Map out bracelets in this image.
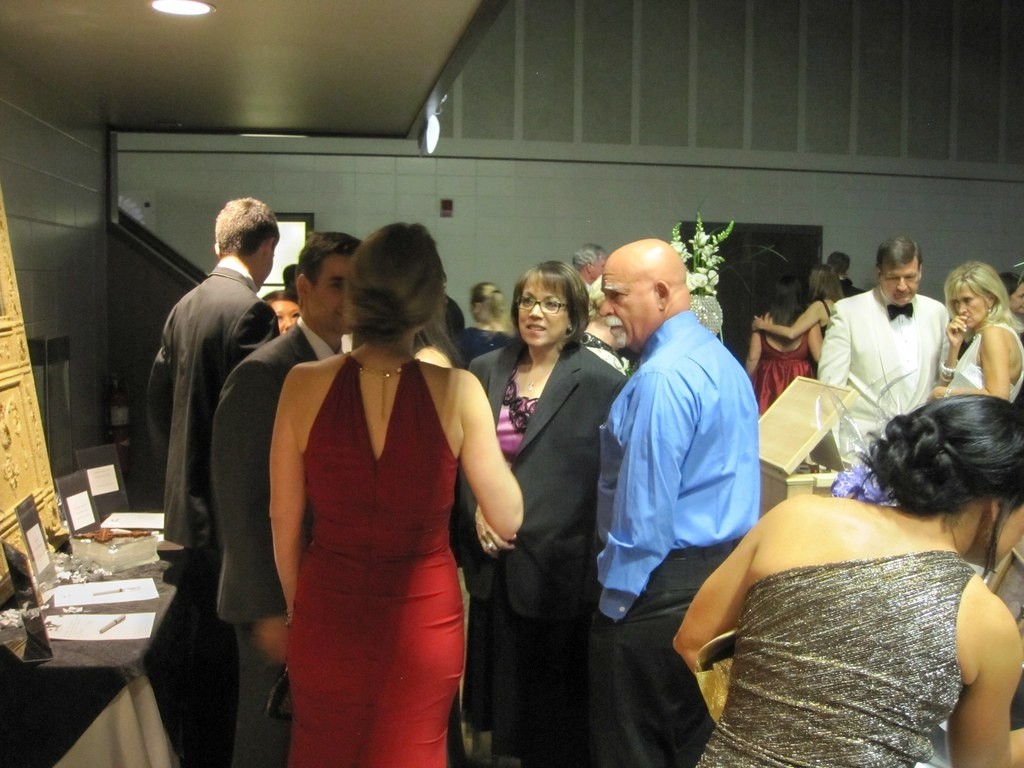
[941,360,958,382]
[944,389,951,397]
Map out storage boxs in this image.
[755,374,865,513]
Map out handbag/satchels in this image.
[693,628,739,725]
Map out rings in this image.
[486,542,494,550]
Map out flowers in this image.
[672,215,734,292]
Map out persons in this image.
[461,261,626,768]
[817,237,952,470]
[927,262,1024,403]
[148,199,279,767]
[415,270,513,768]
[262,265,301,336]
[673,394,1024,768]
[747,252,867,416]
[591,238,760,768]
[958,273,1024,360]
[573,246,627,378]
[214,231,361,768]
[270,223,523,768]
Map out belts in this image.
[667,539,740,559]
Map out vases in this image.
[687,289,726,336]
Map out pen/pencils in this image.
[99,614,126,634]
[93,587,144,597]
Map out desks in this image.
[0,504,184,768]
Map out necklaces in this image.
[526,364,550,391]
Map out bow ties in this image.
[887,302,914,320]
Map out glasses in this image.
[515,295,569,315]
